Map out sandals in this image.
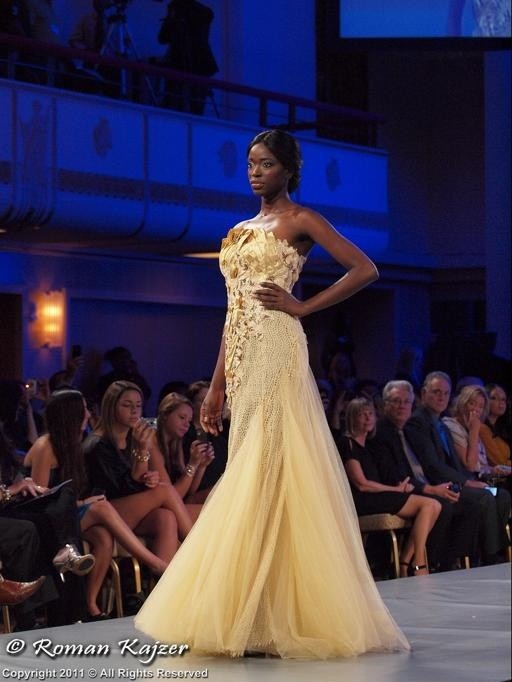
[390,560,427,578]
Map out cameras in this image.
[195,441,210,453]
[140,416,158,430]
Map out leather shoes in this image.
[1,571,48,608]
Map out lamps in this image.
[27,286,67,351]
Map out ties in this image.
[397,429,427,485]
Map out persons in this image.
[0,344,510,629]
[0,1,219,117]
[130,128,409,661]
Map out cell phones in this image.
[449,480,463,493]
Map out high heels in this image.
[53,543,99,584]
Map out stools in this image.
[0,508,512,637]
